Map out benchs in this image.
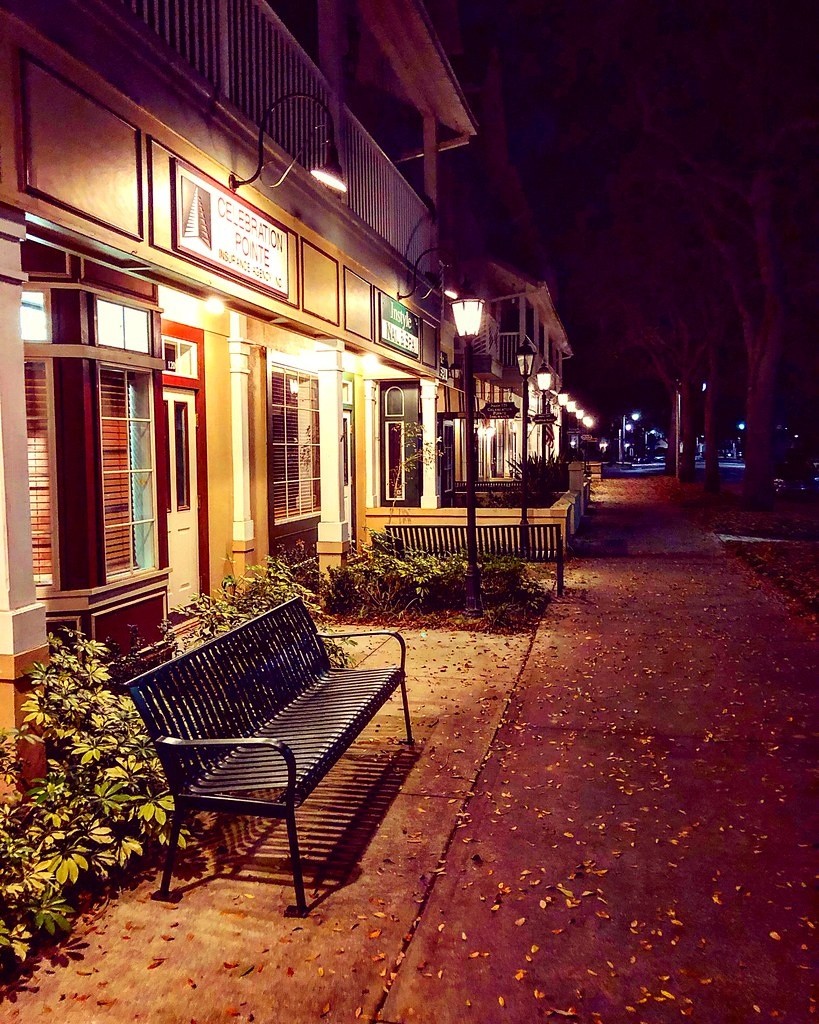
[385,523,568,595]
[123,595,416,917]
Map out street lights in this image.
[536,363,552,468]
[449,288,486,619]
[557,388,594,471]
[514,337,538,560]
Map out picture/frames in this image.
[145,133,301,311]
[421,318,438,370]
[17,46,145,243]
[343,265,374,342]
[373,285,422,363]
[300,236,340,328]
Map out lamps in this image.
[229,92,348,195]
[398,247,494,338]
[448,362,460,380]
[289,379,299,401]
